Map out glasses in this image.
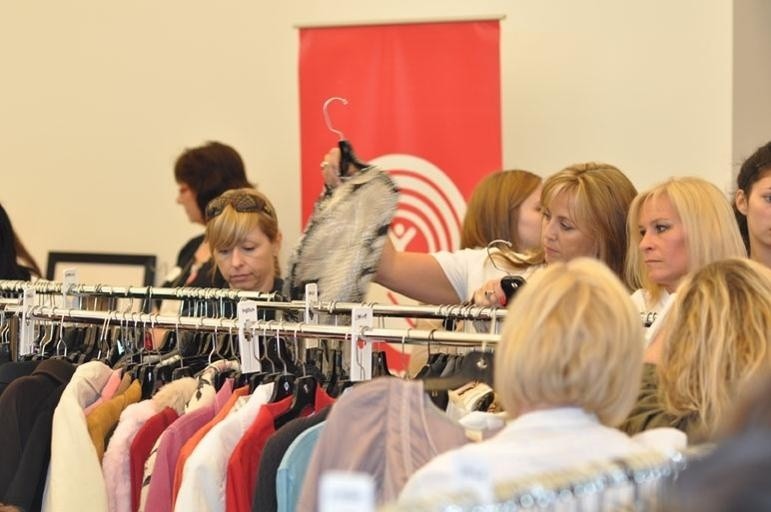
[204,192,275,222]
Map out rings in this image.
[321,162,330,169]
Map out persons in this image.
[1,203,42,300]
[394,261,693,509]
[458,169,545,356]
[321,145,638,311]
[621,176,747,333]
[616,262,771,427]
[151,139,255,305]
[180,188,289,354]
[733,140,771,267]
